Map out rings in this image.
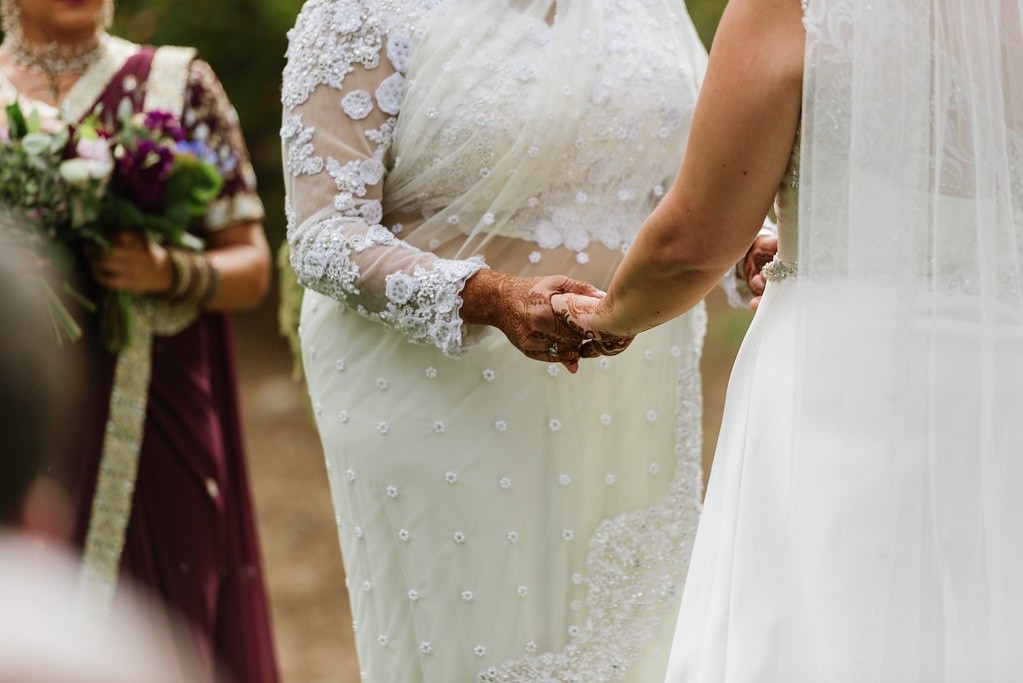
[544,342,560,357]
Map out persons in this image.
[281,0,783,683]
[0,0,281,683]
[0,214,226,682]
[548,0,1021,682]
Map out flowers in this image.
[2,91,240,247]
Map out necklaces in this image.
[1,31,105,73]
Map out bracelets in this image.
[149,248,217,313]
